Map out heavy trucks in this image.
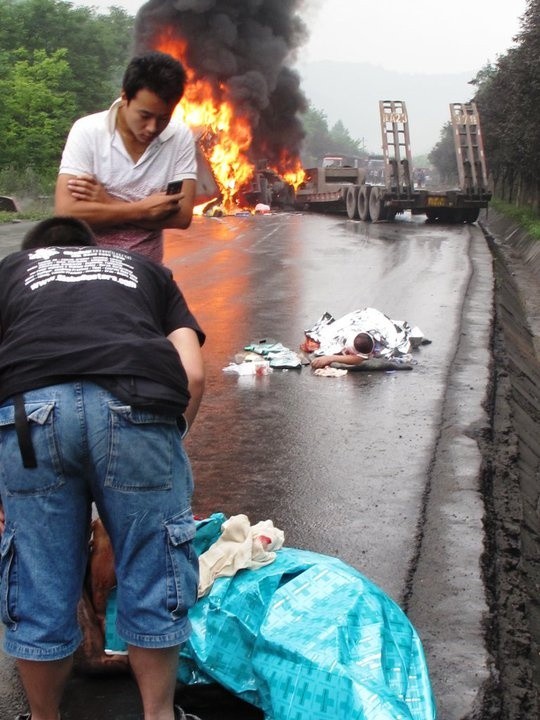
[294,99,492,223]
[365,157,395,185]
[318,152,365,169]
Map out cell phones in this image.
[166,180,183,195]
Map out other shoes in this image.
[15,710,32,720]
[173,703,202,720]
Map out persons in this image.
[52,52,197,267]
[312,307,397,369]
[0,486,435,720]
[0,216,206,720]
[366,165,429,188]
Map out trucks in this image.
[245,157,293,208]
